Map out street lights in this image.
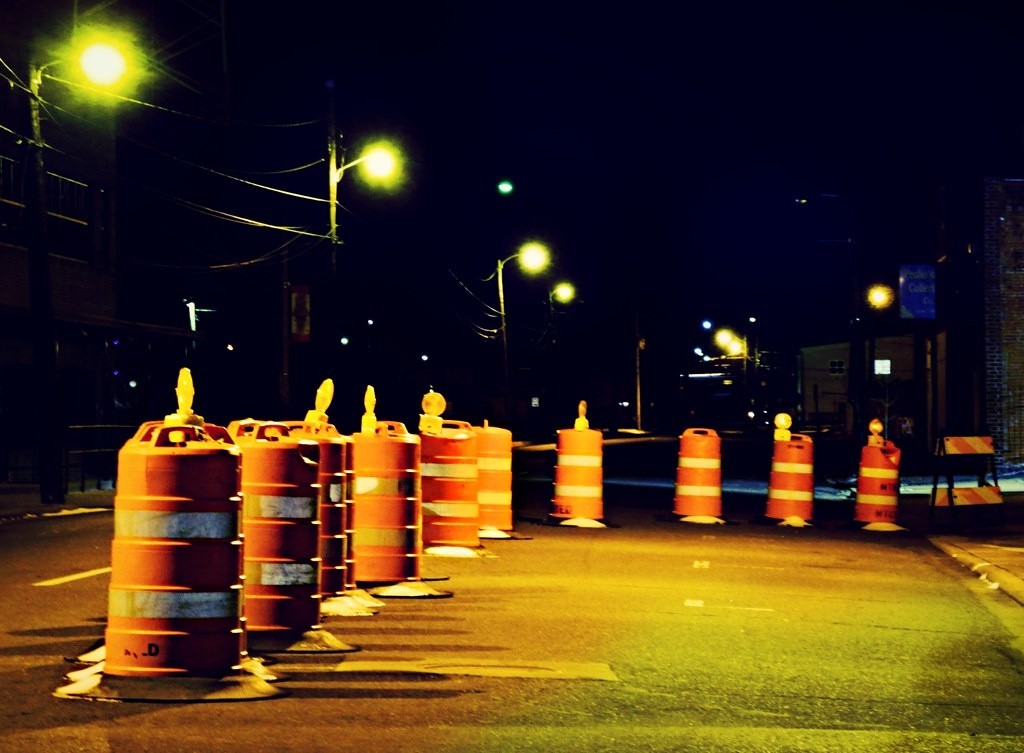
[494,241,553,350]
[326,107,409,248]
[19,21,146,152]
